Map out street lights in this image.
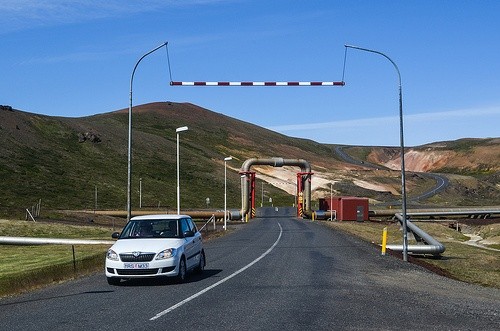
[224,157,233,230]
[176,126,188,215]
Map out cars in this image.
[105,215,207,284]
